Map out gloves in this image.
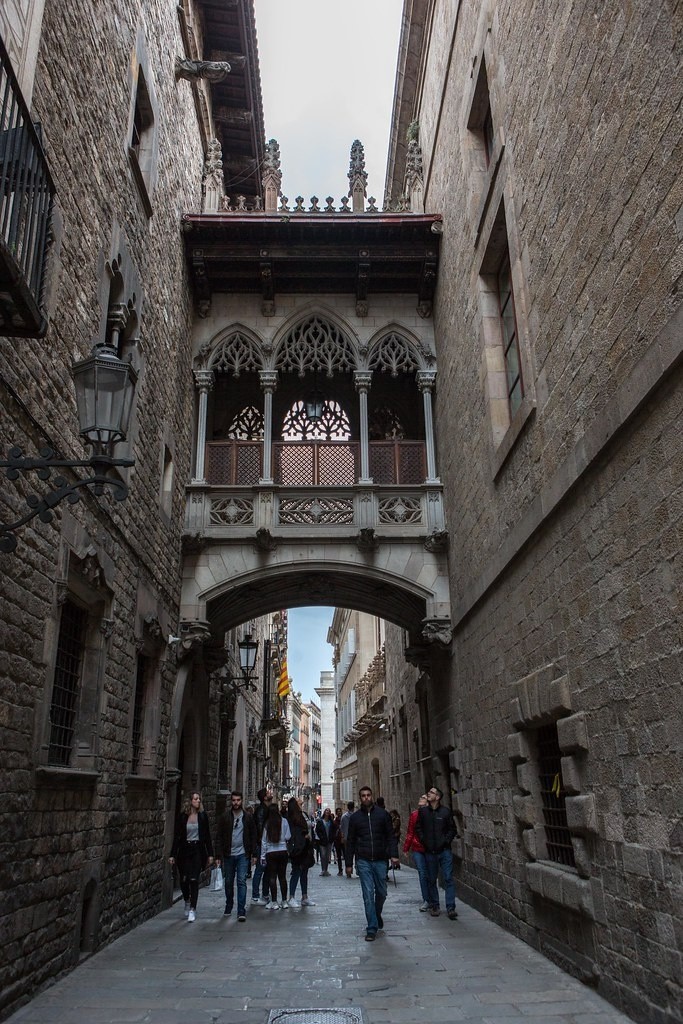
[260,859,267,867]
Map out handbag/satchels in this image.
[208,863,222,892]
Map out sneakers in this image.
[188,910,196,922]
[251,896,316,910]
[184,905,191,916]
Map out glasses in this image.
[235,818,239,827]
[429,791,437,795]
[421,796,428,800]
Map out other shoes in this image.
[376,913,383,929]
[447,906,458,917]
[430,903,440,917]
[365,932,376,941]
[246,873,251,878]
[319,871,331,876]
[419,900,433,912]
[224,905,232,915]
[239,915,247,921]
[347,873,351,878]
[337,871,343,876]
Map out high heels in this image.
[395,864,400,870]
[389,865,395,870]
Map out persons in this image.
[213,791,258,921]
[377,797,401,870]
[168,791,214,922]
[245,788,354,910]
[346,786,400,941]
[403,787,458,917]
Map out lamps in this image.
[0,344,134,557]
[210,636,262,705]
